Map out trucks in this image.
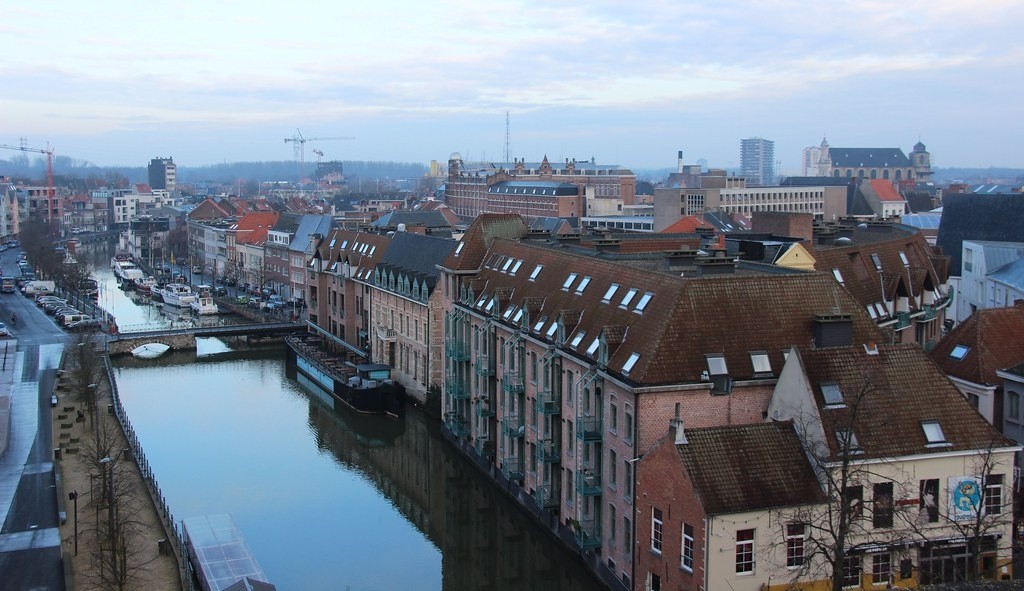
[0,276,15,293]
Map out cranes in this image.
[0,137,53,155]
[283,128,356,182]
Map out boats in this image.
[181,513,276,590]
[283,330,405,418]
[111,249,222,316]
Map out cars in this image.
[33,291,93,328]
[239,282,306,313]
[155,262,187,283]
[191,265,203,274]
[206,284,228,297]
[15,250,36,284]
[0,240,17,253]
[0,322,8,336]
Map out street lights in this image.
[99,455,118,583]
[87,383,99,454]
